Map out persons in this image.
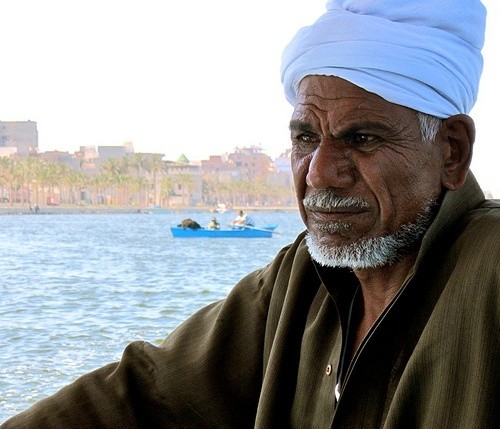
[0,0,499,429]
[208,217,220,229]
[230,209,255,230]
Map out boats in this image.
[137,206,173,215]
[210,204,234,214]
[171,224,277,238]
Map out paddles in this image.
[226,223,281,234]
